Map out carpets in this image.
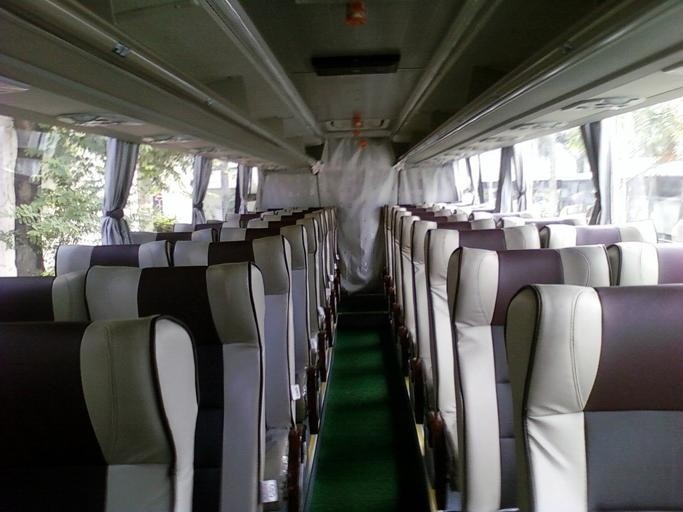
[301,280,437,512]
